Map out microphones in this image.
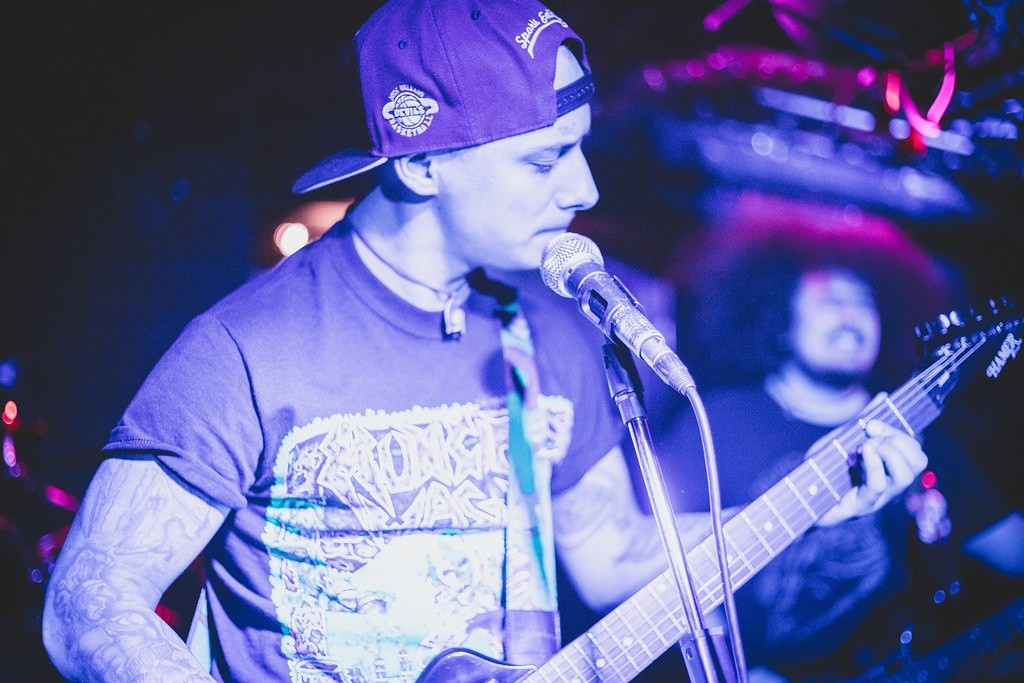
[539,232,696,396]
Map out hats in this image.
[291,0,595,196]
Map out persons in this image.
[36,0,931,682]
[652,267,955,683]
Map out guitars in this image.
[410,293,1023,683]
